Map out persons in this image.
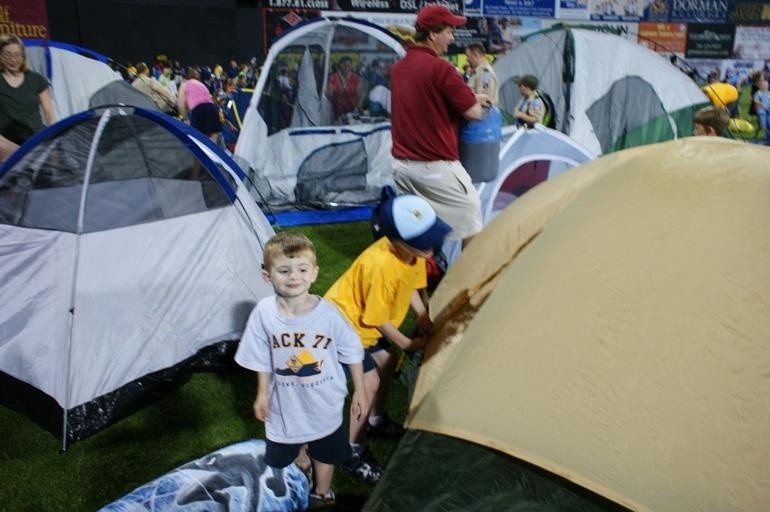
[387,5,493,255]
[111,53,470,153]
[319,191,454,484]
[461,63,473,84]
[0,31,57,164]
[511,74,557,131]
[462,41,500,109]
[669,53,770,143]
[234,226,371,511]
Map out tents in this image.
[491,21,745,158]
[356,136,770,510]
[21,37,188,138]
[432,118,598,275]
[230,14,415,236]
[2,100,277,456]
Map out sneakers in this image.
[343,460,383,483]
[310,493,336,510]
[370,411,405,435]
[294,463,314,490]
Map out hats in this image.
[513,73,538,89]
[414,6,467,30]
[378,195,449,250]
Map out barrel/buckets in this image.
[457,102,502,184]
